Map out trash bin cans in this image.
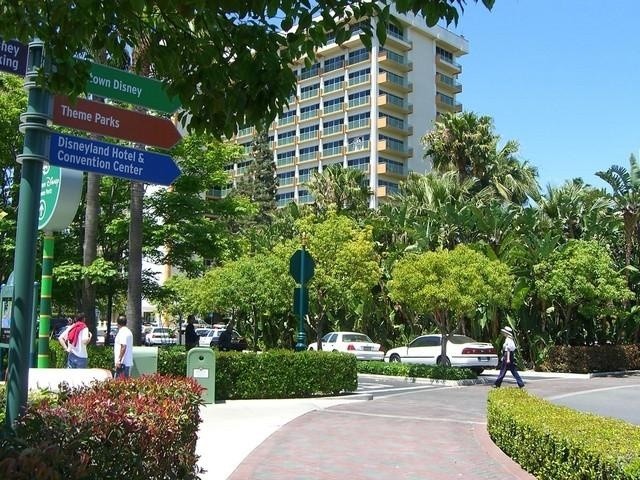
[186,348,215,404]
[130,347,158,377]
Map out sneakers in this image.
[493,385,500,389]
[520,385,526,389]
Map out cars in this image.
[380,332,500,378]
[34,311,250,355]
[305,330,385,361]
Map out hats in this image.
[501,325,513,336]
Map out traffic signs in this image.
[45,87,184,151]
[0,34,29,75]
[38,133,181,191]
[49,50,186,118]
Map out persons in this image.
[58,312,93,368]
[492,326,525,388]
[185,315,200,351]
[218,326,235,352]
[114,316,134,378]
